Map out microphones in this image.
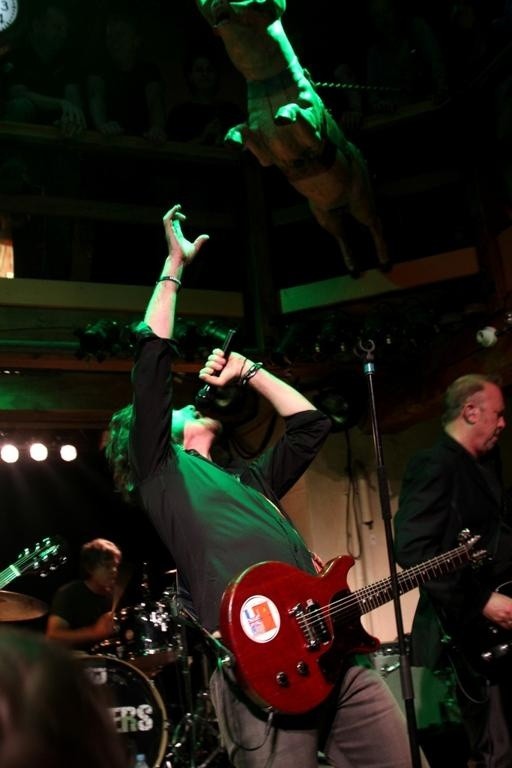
[194,327,241,409]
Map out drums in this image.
[92,602,186,674]
[165,694,234,768]
[69,652,168,768]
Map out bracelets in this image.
[239,357,248,378]
[237,361,264,386]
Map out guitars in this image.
[481,579,512,681]
[216,526,488,718]
[0,536,67,590]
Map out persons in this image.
[0,0,512,293]
[393,366,512,768]
[46,537,124,652]
[100,204,430,768]
